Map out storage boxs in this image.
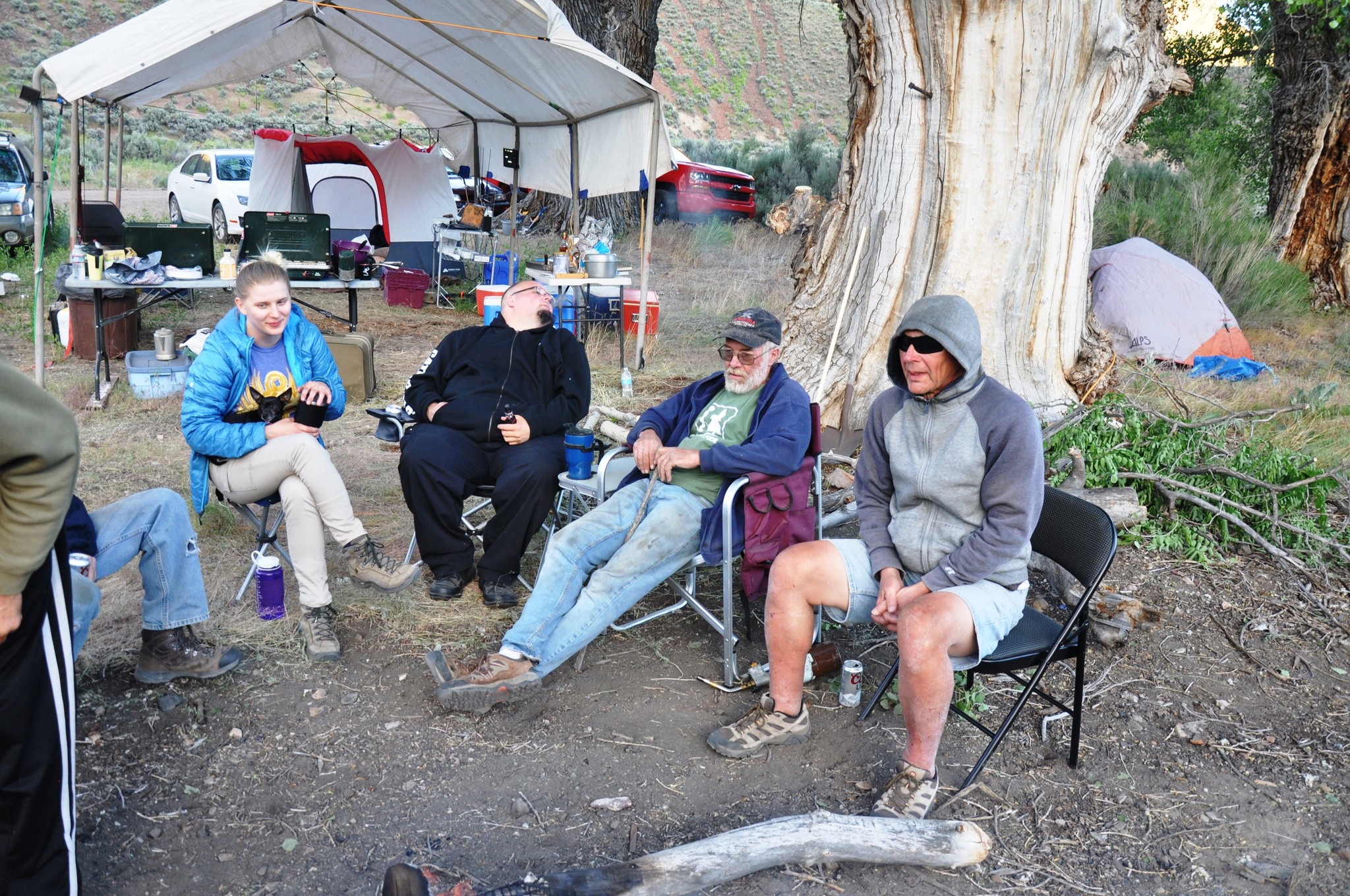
[476,284,659,337]
[236,211,331,279]
[125,351,192,400]
[124,221,216,273]
[423,292,436,303]
[381,266,429,308]
[69,297,139,360]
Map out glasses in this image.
[511,285,557,304]
[896,332,945,354]
[718,344,777,365]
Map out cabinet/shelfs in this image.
[432,224,499,311]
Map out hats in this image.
[712,307,783,346]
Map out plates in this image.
[169,277,202,280]
[1,275,20,281]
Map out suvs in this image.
[0,130,55,247]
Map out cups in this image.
[87,248,103,280]
[358,263,377,280]
[565,423,594,480]
[294,393,328,428]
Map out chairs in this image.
[363,406,559,597]
[858,485,1117,790]
[216,429,325,601]
[557,402,826,687]
[81,200,128,250]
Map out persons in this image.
[706,295,1045,819]
[424,308,814,712]
[397,283,590,608]
[180,261,421,661]
[64,488,243,685]
[1,358,82,896]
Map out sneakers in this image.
[869,758,940,819]
[341,534,421,593]
[134,626,243,683]
[705,692,811,760]
[423,649,490,716]
[435,651,542,713]
[296,603,343,661]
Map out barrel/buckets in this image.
[502,221,518,235]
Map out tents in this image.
[35,0,690,383]
[1087,237,1256,368]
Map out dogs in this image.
[200,385,293,467]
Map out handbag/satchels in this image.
[578,215,614,250]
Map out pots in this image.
[583,253,621,278]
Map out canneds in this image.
[839,659,863,706]
[69,552,90,580]
[384,404,402,419]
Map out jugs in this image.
[154,328,177,360]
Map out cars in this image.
[369,142,509,214]
[166,148,257,242]
[481,144,758,227]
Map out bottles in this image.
[568,235,580,273]
[504,404,516,424]
[251,550,285,621]
[72,245,85,281]
[219,249,237,280]
[621,368,633,398]
[93,239,102,249]
[553,252,569,278]
[559,232,568,252]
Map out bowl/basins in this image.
[331,240,371,263]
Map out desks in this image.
[64,272,381,413]
[525,268,632,371]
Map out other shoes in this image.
[478,576,517,608]
[382,864,430,896]
[428,564,476,601]
